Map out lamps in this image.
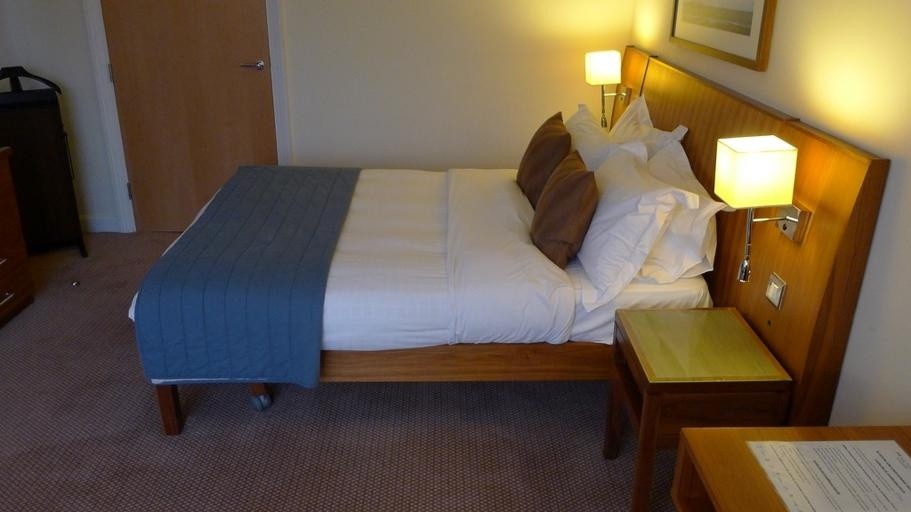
[584,50,633,127]
[713,135,811,284]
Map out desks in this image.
[670,424,909,512]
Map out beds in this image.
[128,45,890,434]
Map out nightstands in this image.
[603,306,796,511]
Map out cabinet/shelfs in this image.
[1,146,35,327]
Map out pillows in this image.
[529,149,597,269]
[608,92,689,162]
[577,146,699,313]
[516,111,571,212]
[633,143,727,284]
[563,104,620,172]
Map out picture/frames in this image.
[669,0,778,72]
